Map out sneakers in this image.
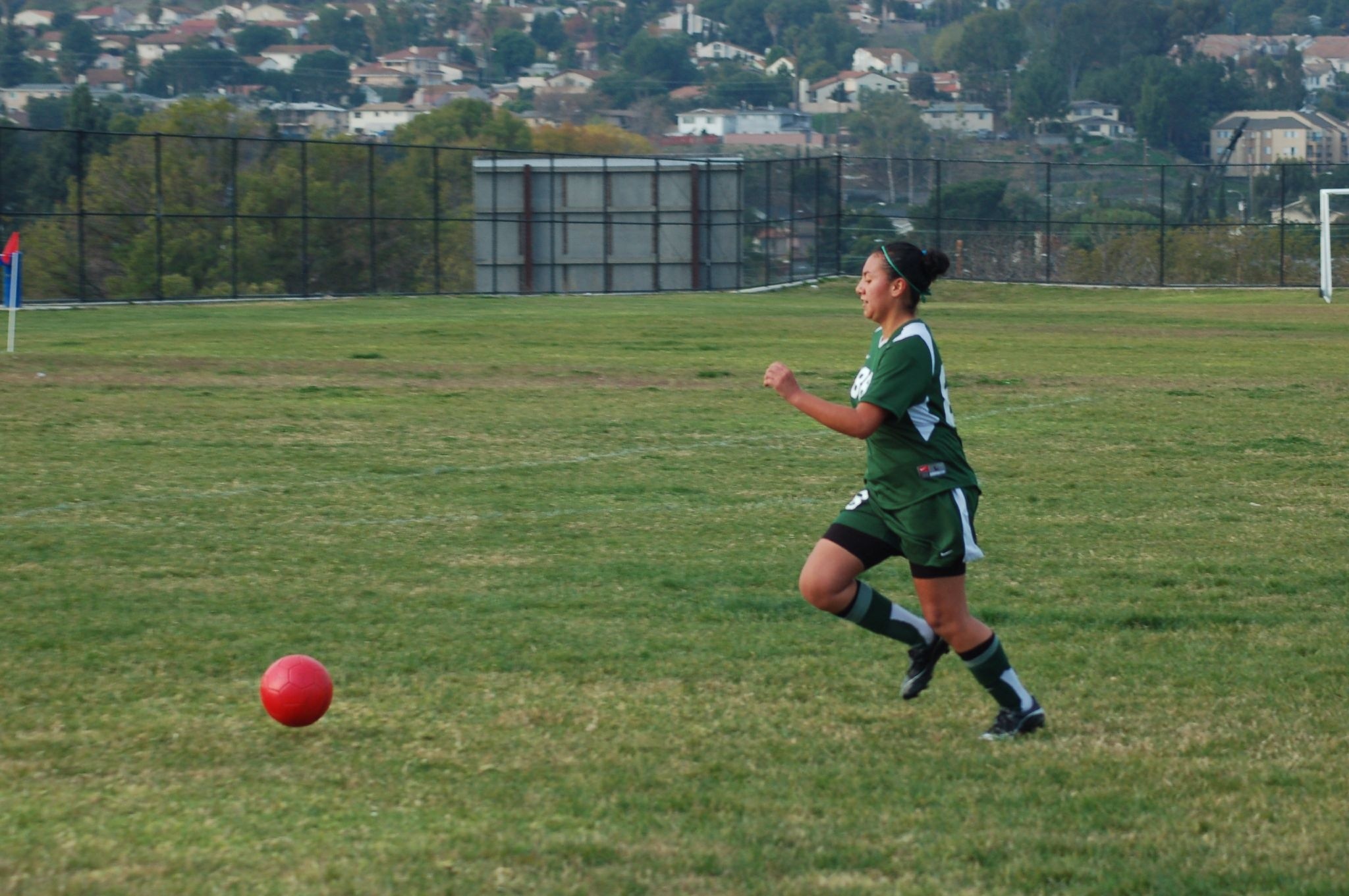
[981,698,1046,742]
[899,635,952,698]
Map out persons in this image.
[764,241,1046,741]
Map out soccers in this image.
[261,655,332,728]
